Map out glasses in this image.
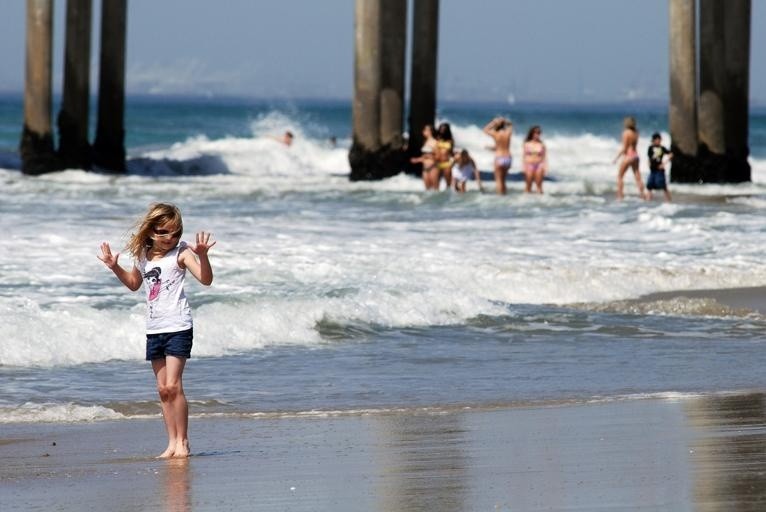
[153,226,181,238]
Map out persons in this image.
[264,130,293,148]
[435,123,455,188]
[483,117,513,196]
[612,116,647,201]
[646,132,675,202]
[409,123,441,191]
[521,125,548,193]
[449,149,484,193]
[162,458,194,511]
[93,201,218,460]
